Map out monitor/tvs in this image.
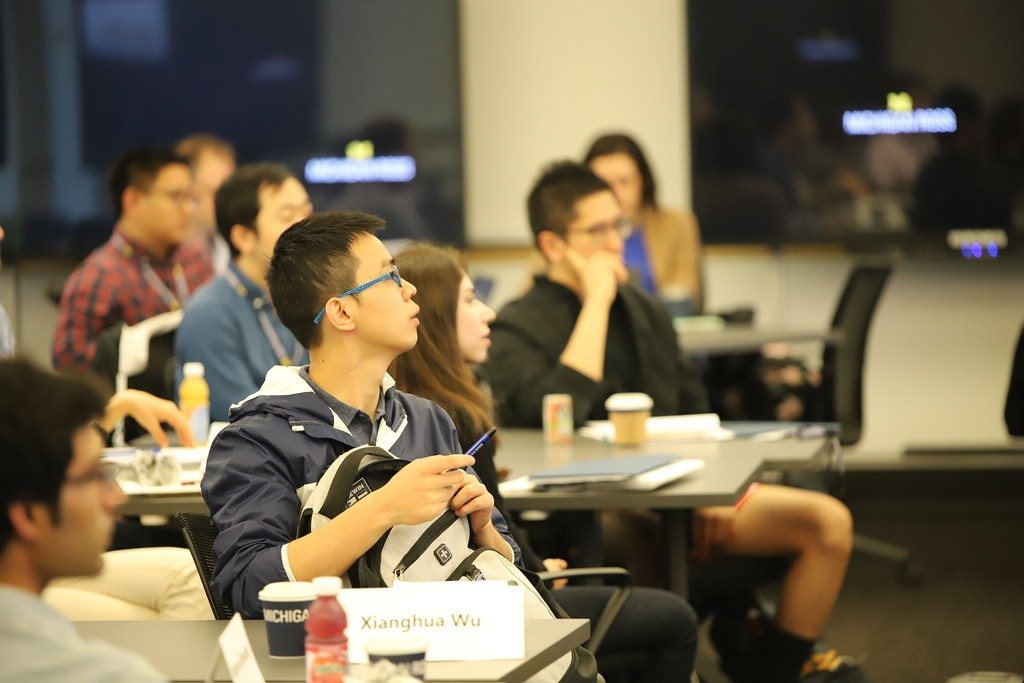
[685,0,1024,262]
[0,0,466,266]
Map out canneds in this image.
[542,393,574,446]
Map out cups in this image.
[258,581,318,660]
[605,391,655,447]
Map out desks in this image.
[717,418,840,470]
[103,429,766,598]
[71,619,591,683]
[678,324,845,418]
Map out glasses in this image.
[313,264,402,326]
[563,217,636,241]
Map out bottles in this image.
[177,361,211,447]
[303,575,349,683]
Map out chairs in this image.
[534,566,630,651]
[174,512,236,620]
[694,258,924,583]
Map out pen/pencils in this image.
[181,479,201,485]
[445,426,497,472]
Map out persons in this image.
[0,353,164,683]
[692,70,1024,240]
[336,114,434,241]
[50,133,314,421]
[581,135,701,316]
[484,160,864,683]
[1004,321,1024,436]
[201,213,602,683]
[393,245,701,683]
[0,226,215,621]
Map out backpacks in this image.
[294,445,596,683]
